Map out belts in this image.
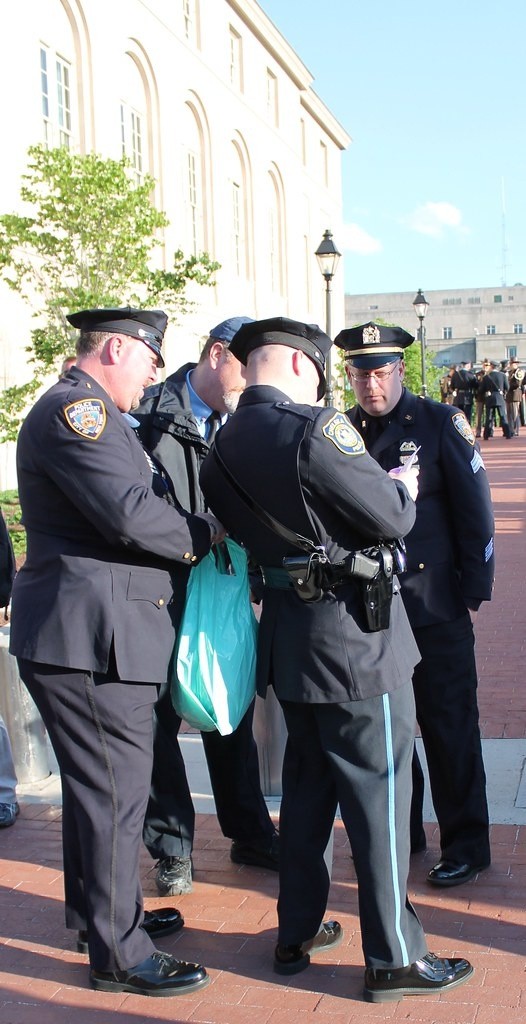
[455,389,470,393]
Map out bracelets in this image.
[203,519,217,546]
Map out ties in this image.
[207,410,221,447]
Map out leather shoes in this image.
[274,920,344,976]
[89,949,212,996]
[427,860,491,886]
[363,951,474,1003]
[77,907,185,952]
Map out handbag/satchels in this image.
[170,536,259,736]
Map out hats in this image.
[229,317,335,402]
[490,361,500,366]
[66,306,169,368]
[211,316,256,341]
[333,321,416,370]
[500,360,509,364]
[509,357,520,363]
[461,360,471,364]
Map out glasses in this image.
[348,362,399,382]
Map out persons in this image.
[130,318,280,896]
[333,321,495,884]
[8,304,228,998]
[439,356,526,440]
[0,514,20,829]
[59,357,76,381]
[198,318,475,1003]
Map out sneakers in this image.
[153,856,195,897]
[230,829,282,872]
[0,802,19,825]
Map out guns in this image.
[344,546,396,632]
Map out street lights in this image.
[413,288,432,402]
[313,229,342,411]
